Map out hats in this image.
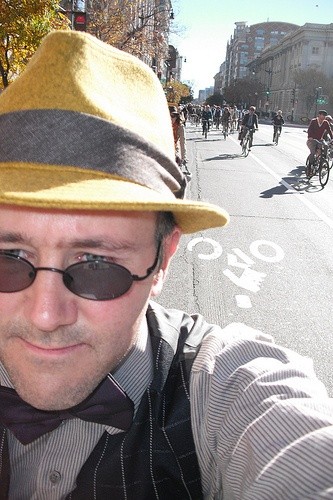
[318,110,328,116]
[0,30,229,234]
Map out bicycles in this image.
[200,118,212,139]
[188,111,245,135]
[238,127,258,157]
[273,123,285,146]
[221,119,231,141]
[306,138,333,186]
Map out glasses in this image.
[0,237,162,301]
[170,114,175,118]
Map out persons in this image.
[0,31,333,500]
[167,102,333,182]
